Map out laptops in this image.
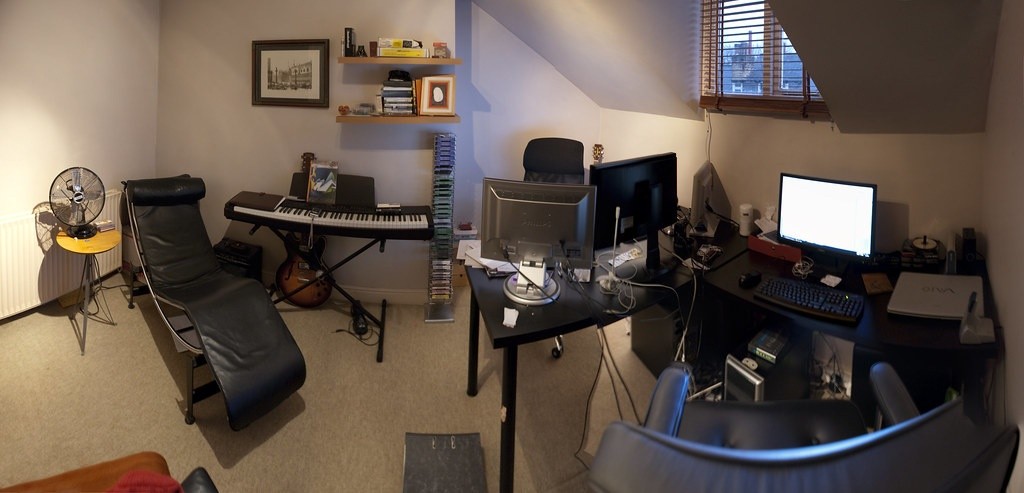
[887,272,985,320]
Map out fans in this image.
[50,167,106,238]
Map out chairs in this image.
[122,172,306,432]
[523,137,586,186]
[586,348,1022,493]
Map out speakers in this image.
[213,237,263,281]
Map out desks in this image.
[458,231,753,492]
[703,243,1006,430]
[54,227,124,351]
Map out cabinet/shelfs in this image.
[333,55,461,123]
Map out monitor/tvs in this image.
[480,177,597,305]
[777,172,878,278]
[689,160,721,237]
[590,151,679,285]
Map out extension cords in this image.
[352,305,368,335]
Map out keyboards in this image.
[754,277,865,325]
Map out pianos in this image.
[222,189,435,365]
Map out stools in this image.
[1,451,220,492]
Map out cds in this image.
[913,238,937,249]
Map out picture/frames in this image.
[421,73,456,117]
[252,39,329,108]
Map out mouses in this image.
[738,271,762,290]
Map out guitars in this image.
[274,151,335,309]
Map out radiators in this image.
[0,187,129,320]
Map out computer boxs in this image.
[724,333,813,403]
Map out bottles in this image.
[432,42,448,58]
[739,204,754,236]
